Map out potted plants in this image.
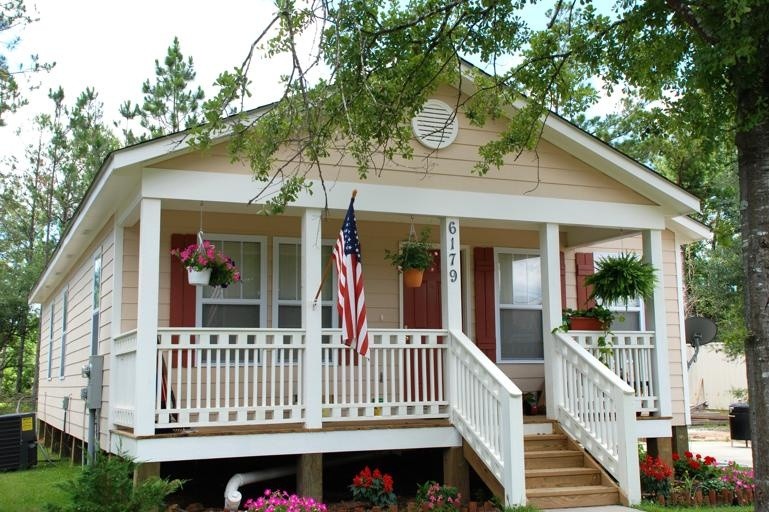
[549,305,626,368]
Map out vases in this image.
[184,264,214,285]
[403,268,424,289]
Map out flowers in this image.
[384,224,438,273]
[170,241,241,287]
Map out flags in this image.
[331,198,372,360]
[157,339,168,409]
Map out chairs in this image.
[730,402,752,447]
